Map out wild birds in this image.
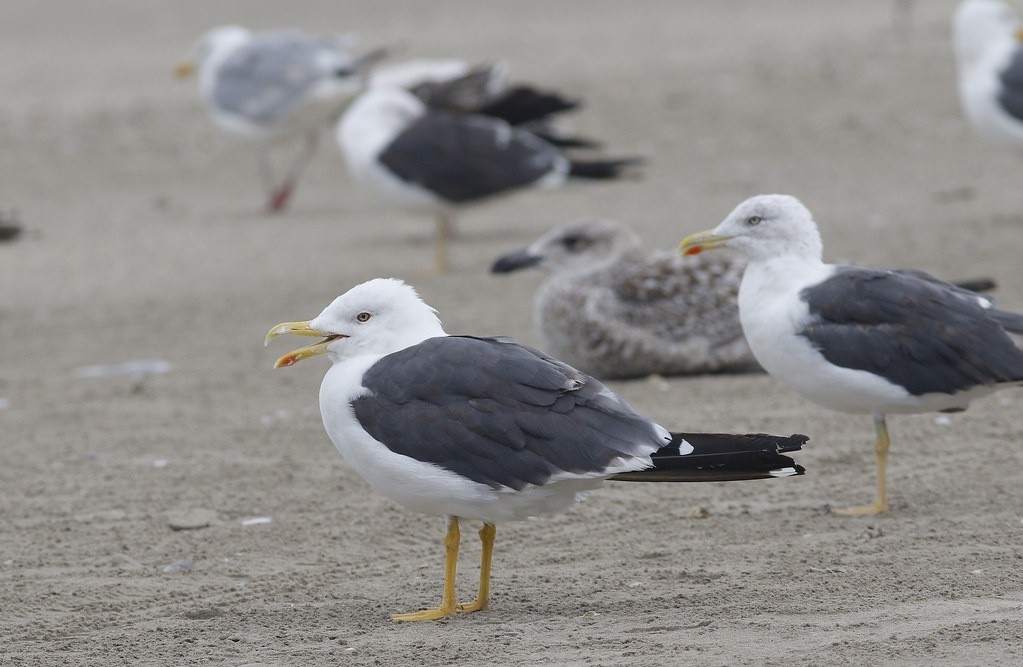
[175,22,652,246]
[952,1,1023,148]
[489,215,998,382]
[264,276,811,623]
[677,192,1023,519]
